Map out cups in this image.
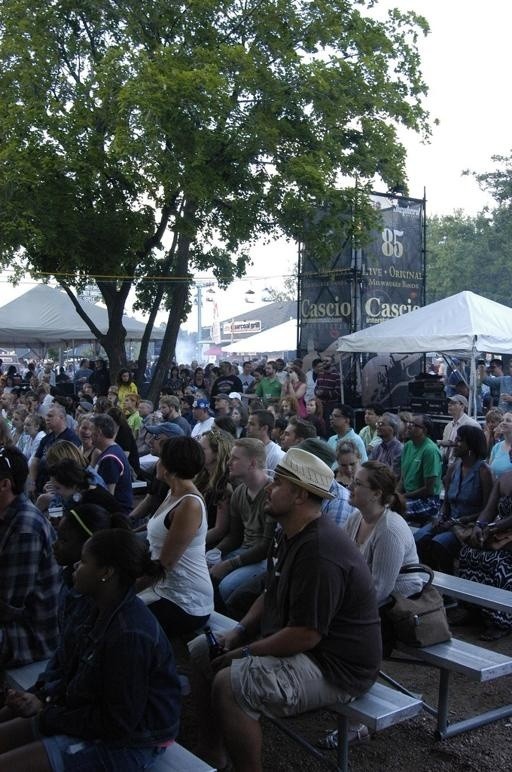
[154,410,162,427]
[207,550,222,565]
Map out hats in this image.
[79,402,94,410]
[181,395,194,405]
[107,385,118,395]
[212,393,229,400]
[288,438,335,469]
[192,399,215,414]
[262,446,337,501]
[145,422,184,437]
[228,392,241,401]
[447,394,467,408]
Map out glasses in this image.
[1,448,11,467]
[406,421,423,429]
[153,432,161,440]
[337,438,356,448]
[351,480,370,489]
[328,414,344,418]
[375,421,392,427]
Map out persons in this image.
[1,354,512,772]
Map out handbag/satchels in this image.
[451,521,512,550]
[386,583,451,648]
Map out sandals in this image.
[315,723,370,749]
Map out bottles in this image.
[205,626,224,659]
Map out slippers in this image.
[479,626,509,642]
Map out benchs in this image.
[418,570,512,622]
[5,656,217,772]
[378,638,512,742]
[204,612,423,771]
[132,480,146,488]
